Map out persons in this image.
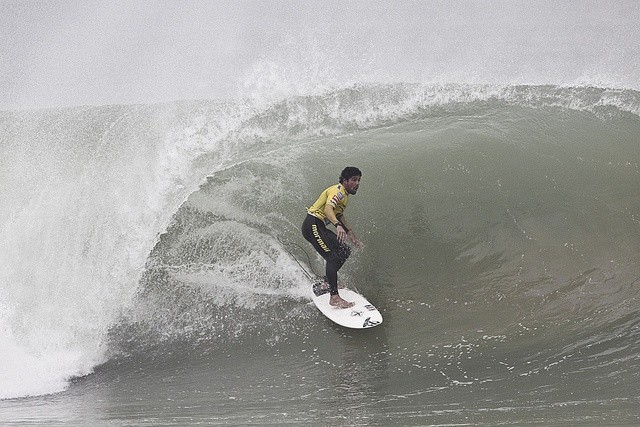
[302,167,365,309]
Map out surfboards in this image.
[311,279,383,329]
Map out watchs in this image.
[334,221,343,228]
